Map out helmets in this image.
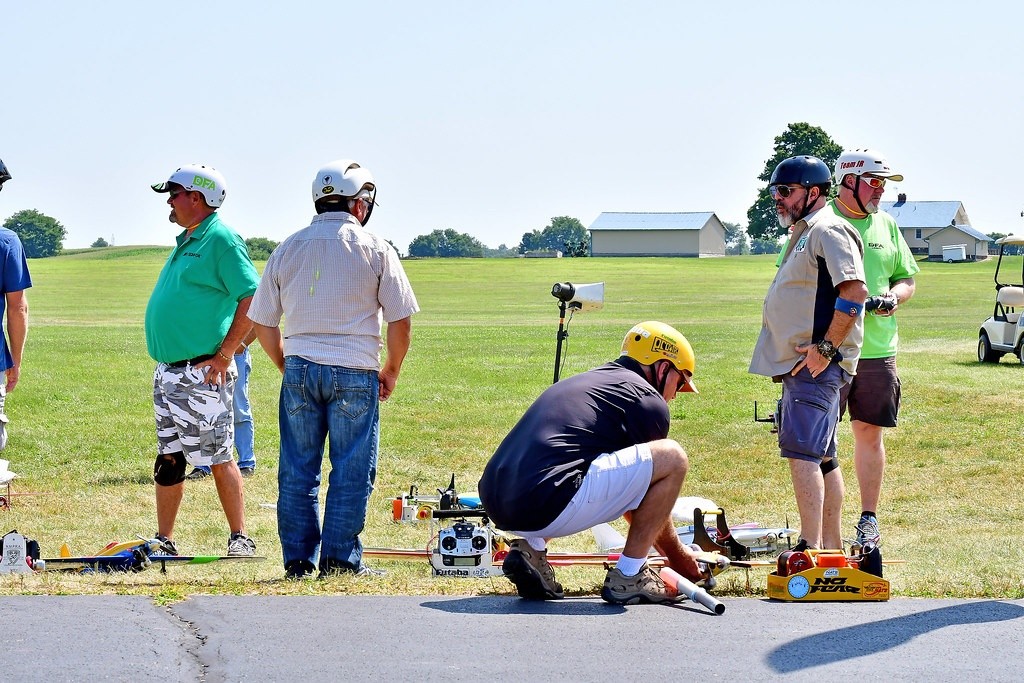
[0,159,12,183]
[620,320,699,396]
[769,155,832,188]
[169,161,228,208]
[835,147,904,187]
[312,159,376,203]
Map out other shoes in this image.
[318,557,386,580]
[789,538,809,552]
[187,468,208,479]
[285,561,315,580]
[239,467,254,477]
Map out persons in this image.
[0,158,32,452]
[144,165,254,557]
[187,330,257,480]
[245,159,421,579]
[776,148,921,550]
[749,156,868,547]
[479,321,705,605]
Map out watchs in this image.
[819,340,838,359]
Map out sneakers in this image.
[227,531,256,556]
[601,561,684,604]
[147,533,178,556]
[502,538,565,599]
[854,514,881,555]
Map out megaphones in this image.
[553,281,605,310]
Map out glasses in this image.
[353,197,372,211]
[850,174,886,188]
[169,189,189,200]
[0,184,3,191]
[769,184,806,198]
[670,365,686,392]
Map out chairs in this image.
[996,287,1024,322]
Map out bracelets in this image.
[241,342,247,347]
[218,351,231,361]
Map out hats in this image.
[151,181,181,193]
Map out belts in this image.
[165,353,218,368]
